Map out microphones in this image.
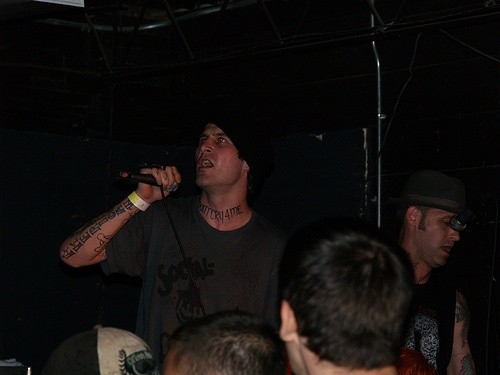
[450,196,487,231]
[122,172,178,192]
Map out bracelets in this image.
[128,191,150,212]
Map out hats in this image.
[389,171,465,214]
[42,324,160,375]
[207,121,249,164]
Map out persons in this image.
[392,168,475,375]
[40,309,289,375]
[59,104,289,375]
[280,230,416,375]
